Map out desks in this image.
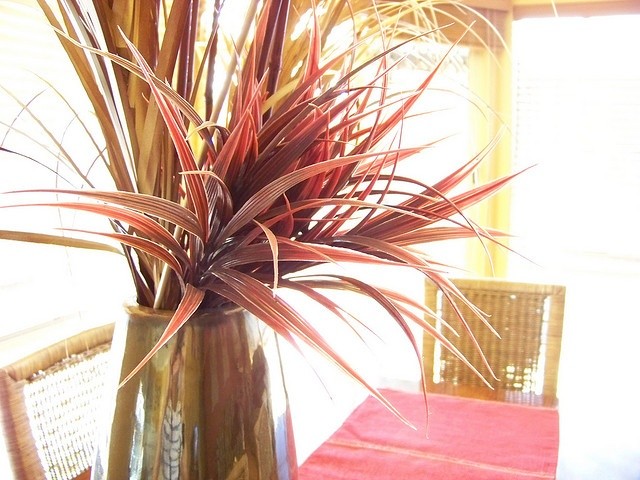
[298,386,560,480]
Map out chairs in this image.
[421,269,566,408]
[0,324,118,479]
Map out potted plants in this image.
[0,0,561,480]
